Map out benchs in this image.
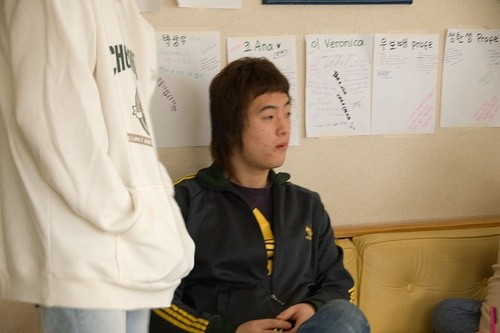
[332,217,500,333]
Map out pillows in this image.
[434,297,482,333]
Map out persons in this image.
[423,241,499,333]
[143,53,371,333]
[0,1,195,333]
